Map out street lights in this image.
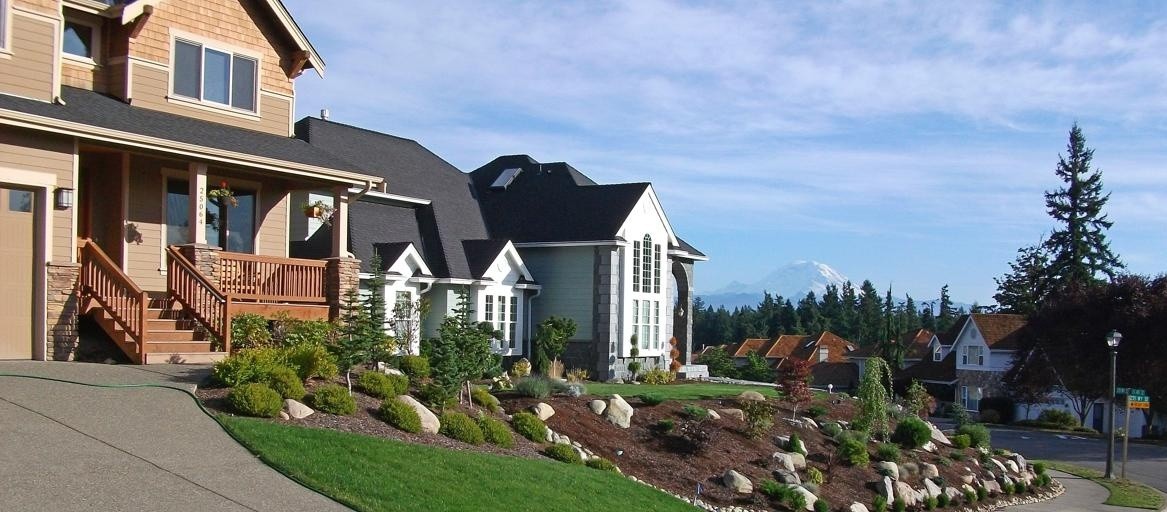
[1105,327,1125,481]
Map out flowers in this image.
[210,181,234,197]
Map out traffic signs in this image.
[1130,396,1151,402]
[1130,389,1146,396]
[1130,403,1150,408]
[1116,386,1130,395]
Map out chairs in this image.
[220,260,261,302]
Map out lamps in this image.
[57,187,76,207]
[674,303,684,316]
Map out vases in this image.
[218,197,232,205]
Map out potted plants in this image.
[300,200,336,226]
